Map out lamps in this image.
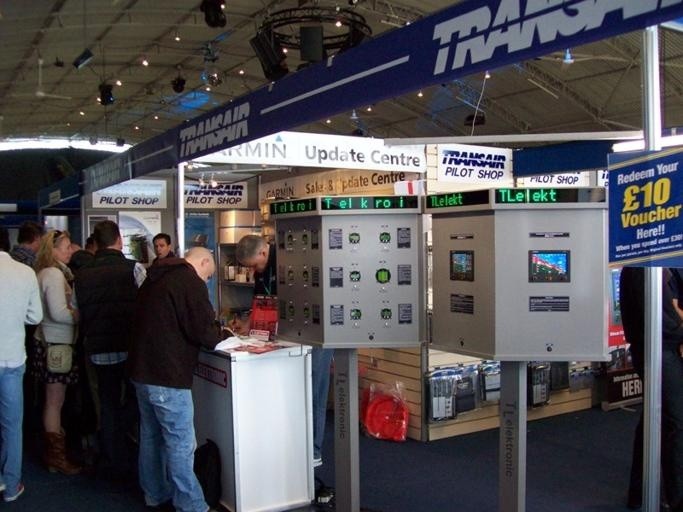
[97,82,113,106]
[71,48,93,69]
[170,69,186,93]
[248,19,373,84]
[200,0,228,29]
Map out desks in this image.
[195,338,317,512]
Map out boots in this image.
[43,428,81,476]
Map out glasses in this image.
[53,230,62,243]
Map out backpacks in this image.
[194,438,222,509]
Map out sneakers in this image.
[143,487,172,507]
[0,481,24,502]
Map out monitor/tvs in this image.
[528,249,571,282]
[449,250,474,282]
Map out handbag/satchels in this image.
[46,344,73,374]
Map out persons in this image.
[228,234,332,467]
[146,233,177,277]
[617,266,682,512]
[124,245,222,511]
[0,220,147,502]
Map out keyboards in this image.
[529,295,570,311]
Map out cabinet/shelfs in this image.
[216,225,257,317]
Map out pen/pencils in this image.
[233,312,238,324]
